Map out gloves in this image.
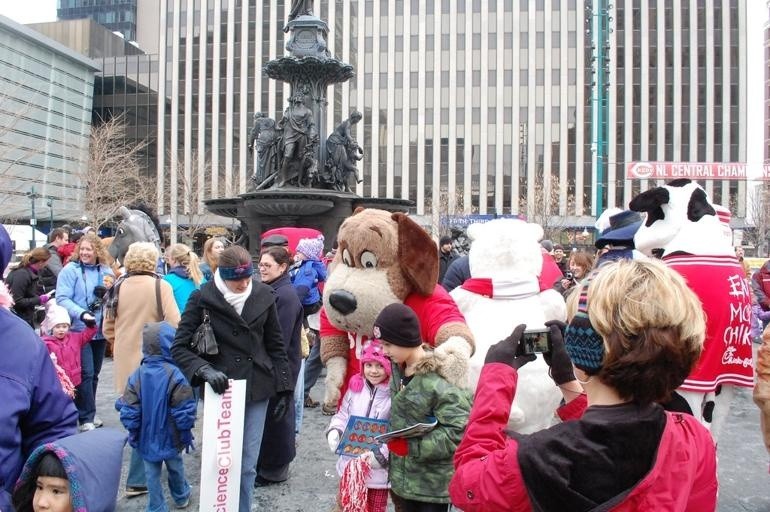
[128,432,140,448]
[484,324,536,370]
[195,365,228,394]
[94,286,107,298]
[274,396,289,422]
[361,439,408,468]
[543,320,576,385]
[80,311,96,328]
[180,431,195,454]
[328,430,340,454]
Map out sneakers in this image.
[307,331,316,347]
[126,486,148,497]
[80,416,103,433]
[304,396,320,408]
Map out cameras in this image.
[88,301,100,311]
[516,330,553,357]
[564,271,575,280]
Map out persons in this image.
[448,254,721,510]
[1,223,475,512]
[734,244,750,278]
[750,259,770,316]
[750,318,770,454]
[277,90,319,190]
[328,109,363,193]
[248,110,278,186]
[346,135,364,187]
[542,238,596,301]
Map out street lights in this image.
[580,227,590,248]
[24,184,38,250]
[44,196,55,231]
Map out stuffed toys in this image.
[624,177,757,431]
[317,203,481,413]
[447,215,570,435]
[106,203,164,265]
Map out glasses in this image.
[258,263,280,268]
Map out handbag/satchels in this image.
[188,323,219,361]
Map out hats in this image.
[373,303,422,347]
[296,235,325,258]
[261,235,288,246]
[47,306,70,330]
[541,240,564,252]
[595,210,644,249]
[440,236,452,246]
[349,339,391,392]
